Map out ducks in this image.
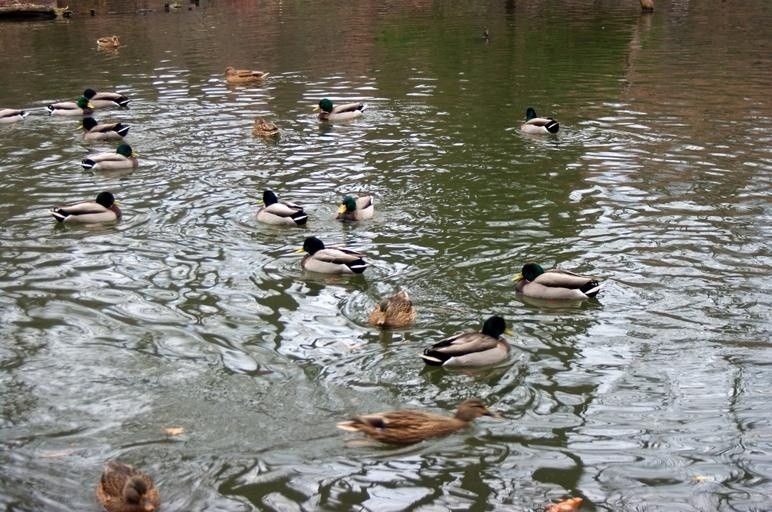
[511,261,606,301]
[414,315,516,368]
[164,2,183,10]
[0,107,30,125]
[219,65,269,85]
[311,98,370,121]
[76,116,132,141]
[96,459,161,511]
[250,118,281,138]
[519,106,560,136]
[80,88,131,109]
[295,236,371,275]
[336,396,500,444]
[74,143,140,171]
[42,96,94,117]
[253,189,309,227]
[94,34,121,49]
[369,286,416,329]
[48,191,124,225]
[335,194,375,221]
